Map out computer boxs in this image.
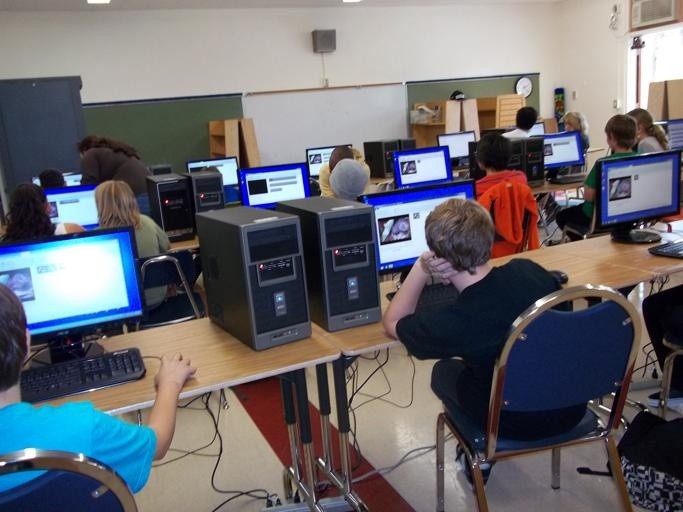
[363,138,416,179]
[468,127,545,188]
[143,164,225,243]
[194,205,312,351]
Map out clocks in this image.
[514,76,533,100]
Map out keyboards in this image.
[550,174,587,185]
[648,241,683,259]
[387,281,461,310]
[20,347,146,403]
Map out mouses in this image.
[548,269,568,284]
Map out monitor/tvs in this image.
[32,171,83,189]
[526,121,546,138]
[530,131,584,178]
[1,225,147,368]
[393,145,455,188]
[39,183,112,232]
[663,118,683,153]
[306,144,355,179]
[597,150,680,244]
[277,196,382,333]
[186,156,242,205]
[237,161,311,211]
[437,130,478,168]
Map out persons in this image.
[2,183,84,244]
[383,198,587,491]
[2,284,198,495]
[626,109,669,153]
[474,132,530,259]
[76,135,151,216]
[94,180,171,310]
[558,112,589,145]
[556,115,642,241]
[500,107,538,140]
[38,169,65,189]
[319,146,371,199]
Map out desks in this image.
[29,315,340,511]
[312,247,654,510]
[544,220,681,413]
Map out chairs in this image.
[0,142,683,512]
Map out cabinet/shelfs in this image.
[459,95,483,144]
[643,80,667,124]
[667,78,682,123]
[205,118,242,169]
[236,115,264,172]
[409,97,462,150]
[476,90,525,132]
